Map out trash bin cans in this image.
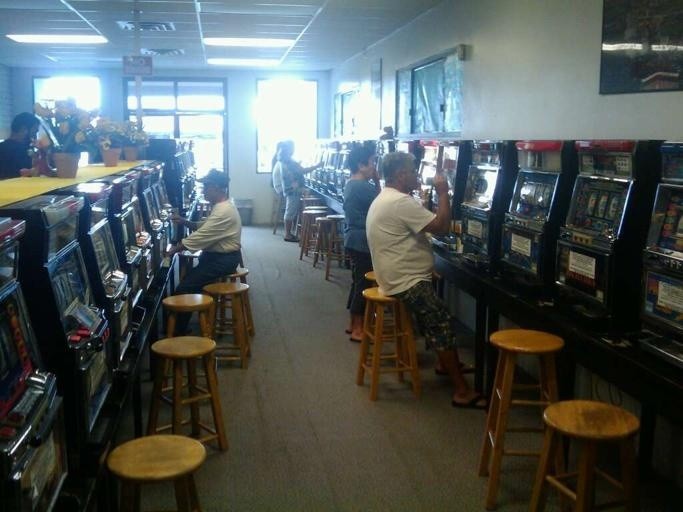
[238,208,252,226]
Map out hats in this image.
[196,169,232,190]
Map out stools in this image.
[162,293,219,395]
[477,329,572,512]
[364,270,410,369]
[529,399,641,512]
[199,200,210,220]
[215,267,255,345]
[201,282,252,369]
[356,287,422,401]
[107,433,206,512]
[273,185,353,281]
[180,250,202,274]
[146,336,229,452]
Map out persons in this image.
[273,160,283,196]
[365,151,491,409]
[270,141,282,187]
[277,140,324,242]
[0,113,40,181]
[343,146,381,345]
[163,169,242,337]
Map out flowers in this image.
[92,119,151,150]
[34,96,99,153]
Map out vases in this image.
[124,147,137,161]
[100,147,121,167]
[54,152,81,178]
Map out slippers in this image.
[449,393,491,410]
[282,236,300,242]
[349,338,376,345]
[345,328,354,334]
[433,363,474,376]
